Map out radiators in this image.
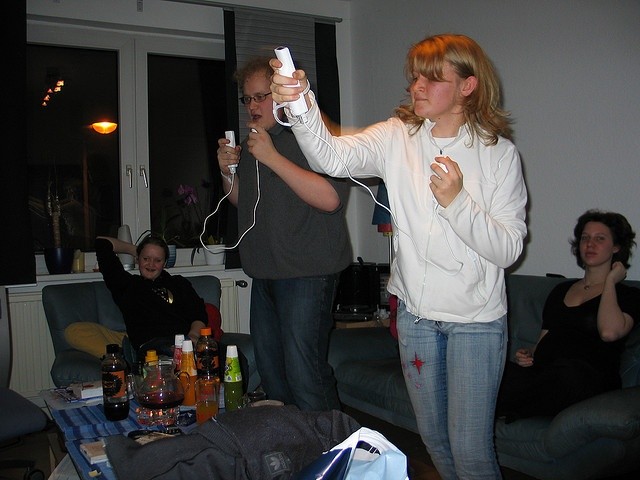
[8,280,248,408]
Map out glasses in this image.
[239,92,271,105]
[147,280,173,304]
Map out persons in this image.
[95,234,249,394]
[217,57,351,412]
[501,212,636,423]
[269,34,528,480]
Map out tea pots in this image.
[129,359,191,411]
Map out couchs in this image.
[40,277,261,393]
[327,275,639,480]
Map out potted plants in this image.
[135,210,184,268]
[44,157,75,274]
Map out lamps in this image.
[373,183,394,268]
[73,97,118,140]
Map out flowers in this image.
[179,183,228,265]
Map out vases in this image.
[204,246,224,265]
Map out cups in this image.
[245,390,266,403]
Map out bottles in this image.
[195,328,220,376]
[180,338,196,405]
[224,345,244,413]
[100,344,129,421]
[195,368,219,425]
[173,334,184,366]
[144,350,158,363]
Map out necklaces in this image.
[582,279,606,290]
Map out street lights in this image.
[84,114,118,249]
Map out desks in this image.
[44,383,224,480]
[334,320,391,330]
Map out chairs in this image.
[0,288,49,478]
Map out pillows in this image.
[64,324,127,359]
[208,303,224,338]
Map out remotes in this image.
[54,388,78,403]
[127,428,182,441]
